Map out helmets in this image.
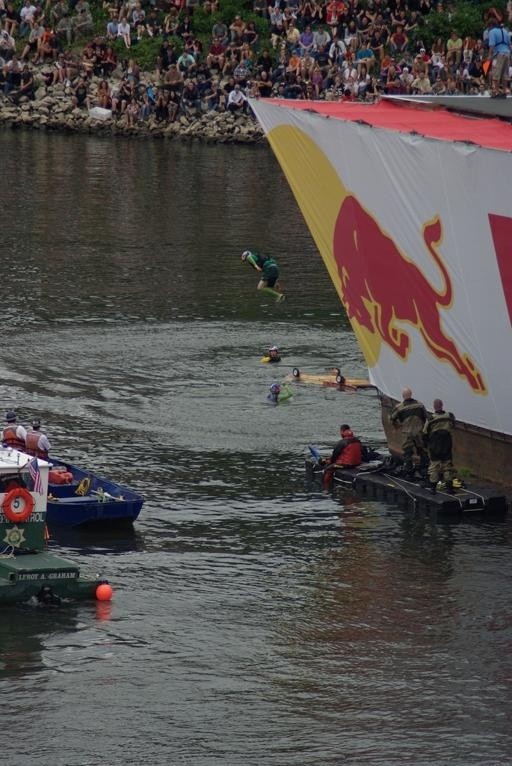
[6,411,17,422]
[271,383,282,393]
[269,345,278,351]
[241,250,250,263]
[31,417,40,428]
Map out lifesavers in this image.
[2,488,34,522]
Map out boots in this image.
[447,482,455,494]
[430,483,437,495]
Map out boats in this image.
[286,369,371,392]
[245,97,512,490]
[305,446,509,524]
[0,443,145,610]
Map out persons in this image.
[260,345,346,403]
[387,388,456,495]
[1,411,52,461]
[0,0,512,128]
[322,424,362,495]
[241,250,286,305]
